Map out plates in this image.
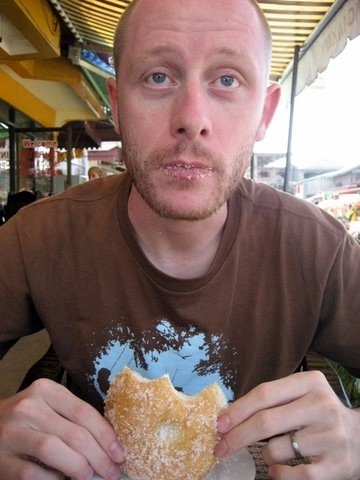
[68,446,257,480]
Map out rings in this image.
[289,430,305,460]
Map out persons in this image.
[2,1,359,479]
[88,166,104,182]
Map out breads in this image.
[102,365,228,479]
